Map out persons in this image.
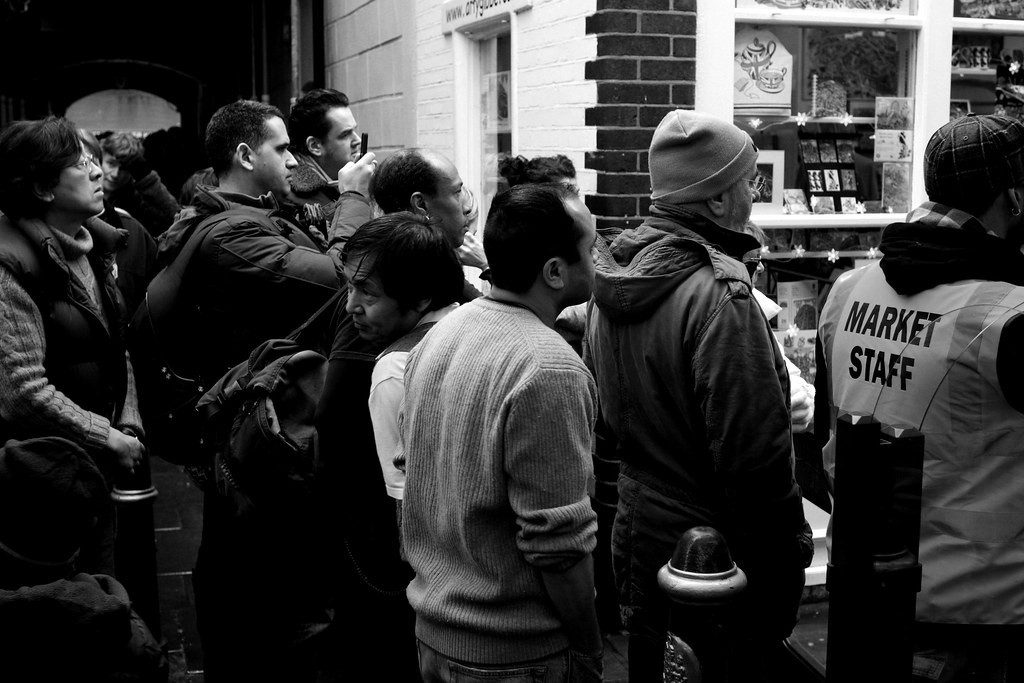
[587,110,813,683]
[814,113,1022,683]
[1,87,380,682]
[314,158,618,683]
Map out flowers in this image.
[1009,60,1020,75]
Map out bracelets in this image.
[340,190,365,198]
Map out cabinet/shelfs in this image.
[729,0,922,256]
[949,0,1024,122]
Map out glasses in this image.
[740,175,766,191]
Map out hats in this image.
[648,110,759,204]
[0,437,105,566]
[924,112,1024,200]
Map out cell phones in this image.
[360,133,369,159]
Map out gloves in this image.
[116,154,151,181]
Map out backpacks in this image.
[195,283,359,528]
[129,210,274,467]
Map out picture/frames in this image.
[749,150,784,217]
